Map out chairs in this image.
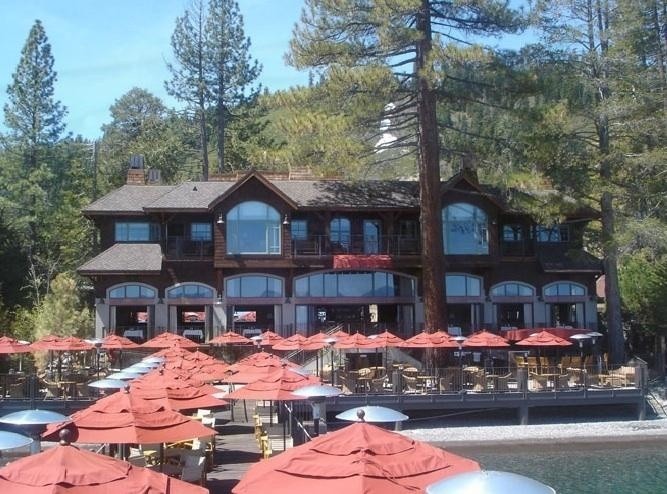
[127,408,216,486]
[516,355,594,377]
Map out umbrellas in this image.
[461,329,511,387]
[515,328,574,376]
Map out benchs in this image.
[9,378,100,402]
